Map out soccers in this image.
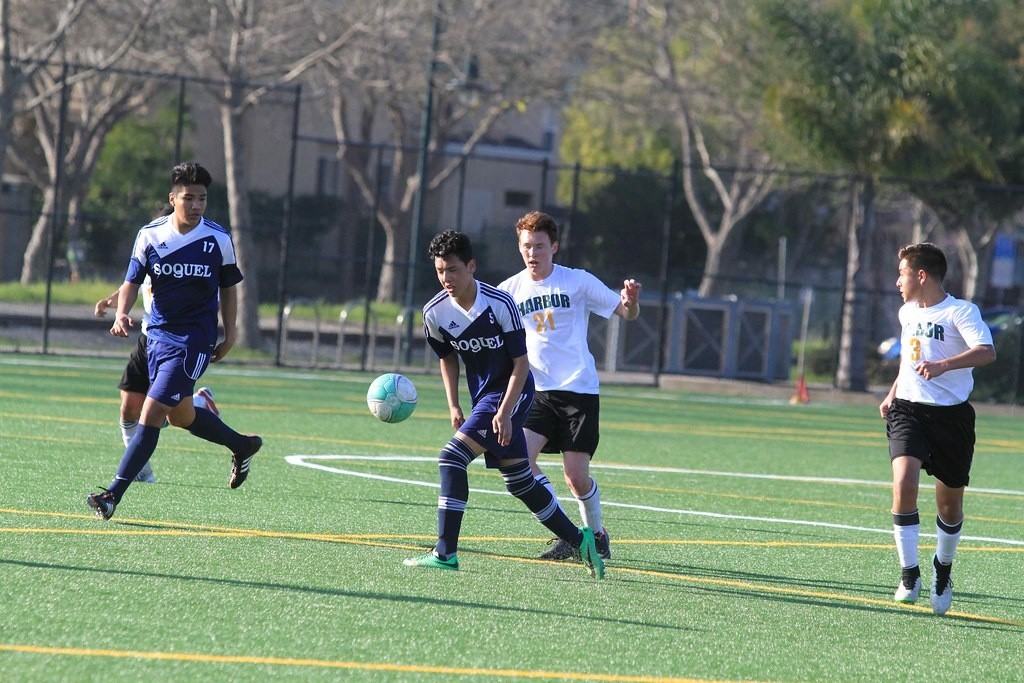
[366,373,419,424]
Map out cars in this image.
[979,303,1024,338]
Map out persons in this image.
[879,243,997,616]
[492,211,640,559]
[84,163,267,523]
[400,227,607,576]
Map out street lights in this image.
[399,0,488,369]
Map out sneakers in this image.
[572,527,605,582]
[593,526,611,559]
[930,554,954,615]
[133,471,155,484]
[87,486,115,519]
[229,435,262,489]
[198,387,220,419]
[537,538,577,563]
[403,548,458,571]
[895,575,922,604]
